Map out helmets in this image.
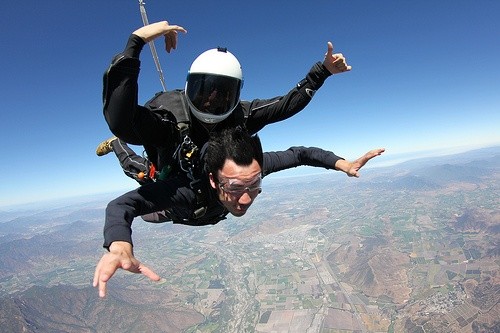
[183,48,244,123]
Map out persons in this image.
[103,19,353,174]
[90,136,386,299]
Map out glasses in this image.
[217,179,262,196]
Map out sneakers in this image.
[96,136,119,156]
